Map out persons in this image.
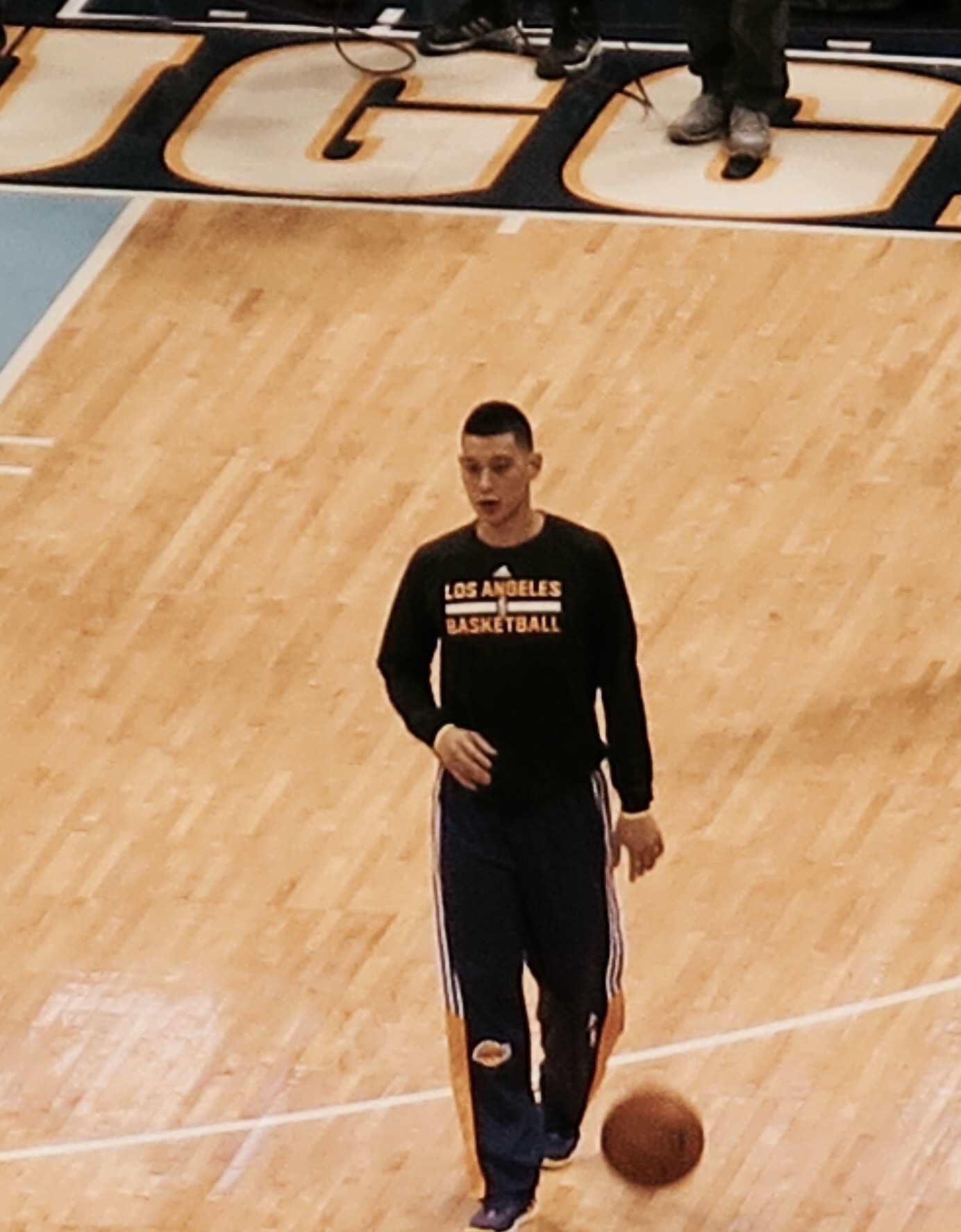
[408,2,607,81]
[376,398,668,1231]
[662,0,796,162]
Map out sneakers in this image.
[540,1123,580,1168]
[415,6,601,81]
[466,1186,540,1232]
[665,95,772,160]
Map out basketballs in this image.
[600,1090,706,1186]
